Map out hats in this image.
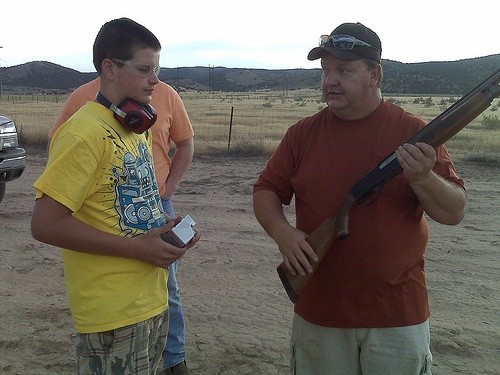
[307,22,382,64]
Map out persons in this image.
[28,17,203,374]
[252,21,469,375]
[48,69,195,375]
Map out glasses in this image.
[319,35,381,53]
[110,57,160,78]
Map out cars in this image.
[0,116,26,203]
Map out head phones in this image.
[97,92,158,135]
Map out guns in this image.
[276,70,500,304]
